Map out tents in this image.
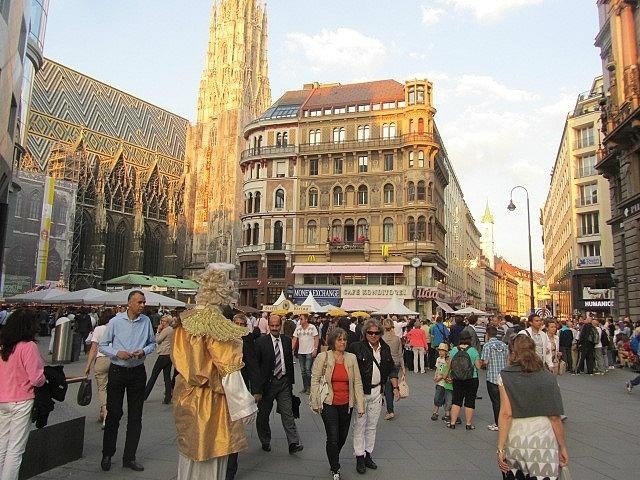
[447,305,486,316]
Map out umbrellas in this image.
[272,290,419,315]
[5,287,187,308]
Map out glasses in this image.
[336,338,348,343]
[367,330,382,335]
[301,318,304,320]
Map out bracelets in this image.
[497,448,506,455]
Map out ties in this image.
[274,339,282,380]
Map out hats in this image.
[460,330,473,338]
[438,343,448,352]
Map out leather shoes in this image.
[124,457,144,471]
[364,452,377,469]
[101,454,111,471]
[262,442,270,451]
[289,443,303,454]
[356,455,366,474]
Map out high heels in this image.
[466,425,476,430]
[450,424,455,428]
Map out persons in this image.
[503,315,513,335]
[443,331,484,430]
[557,320,573,373]
[592,320,604,373]
[503,316,524,345]
[443,316,452,331]
[577,317,599,376]
[484,317,505,343]
[606,316,615,349]
[624,321,640,395]
[465,318,475,331]
[0,308,9,325]
[429,321,439,371]
[481,327,509,431]
[517,314,555,375]
[441,356,462,424]
[613,323,620,348]
[145,314,174,404]
[249,315,304,455]
[430,342,448,421]
[292,314,319,395]
[97,290,156,471]
[567,321,579,375]
[313,314,323,340]
[256,312,270,335]
[231,313,252,395]
[391,315,408,341]
[545,320,566,389]
[327,317,354,352]
[431,316,450,358]
[0,307,46,480]
[381,319,409,420]
[602,327,610,369]
[348,319,401,474]
[309,328,365,480]
[449,315,481,352]
[496,334,569,480]
[407,321,428,374]
[172,262,258,480]
[618,337,638,367]
[621,318,631,337]
[84,308,120,430]
[349,317,357,333]
[420,321,431,368]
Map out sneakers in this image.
[456,417,462,424]
[305,391,310,395]
[562,414,568,421]
[475,396,482,399]
[625,380,633,394]
[431,413,439,421]
[299,391,304,394]
[443,417,450,424]
[331,469,340,480]
[488,424,498,431]
[385,413,394,419]
[161,401,170,404]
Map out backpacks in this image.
[587,325,598,343]
[600,327,609,347]
[450,345,474,381]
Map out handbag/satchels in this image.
[253,318,260,338]
[558,357,566,375]
[308,351,330,414]
[560,465,573,479]
[77,372,93,406]
[398,374,409,397]
[276,395,300,419]
